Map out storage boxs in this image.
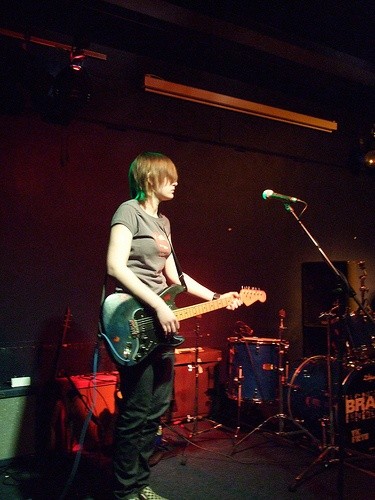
[163,347,222,423]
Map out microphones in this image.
[262,190,303,203]
[237,321,254,334]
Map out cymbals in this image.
[302,321,329,327]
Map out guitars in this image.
[99,284,266,367]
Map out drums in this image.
[221,335,288,405]
[330,311,373,358]
[284,353,375,443]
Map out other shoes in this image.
[127,487,168,500]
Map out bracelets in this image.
[212,293,220,301]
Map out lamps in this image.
[70,47,83,71]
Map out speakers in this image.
[168,348,221,423]
[0,373,119,467]
[299,259,351,328]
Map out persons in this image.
[105,151,245,500]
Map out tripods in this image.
[169,200,375,482]
